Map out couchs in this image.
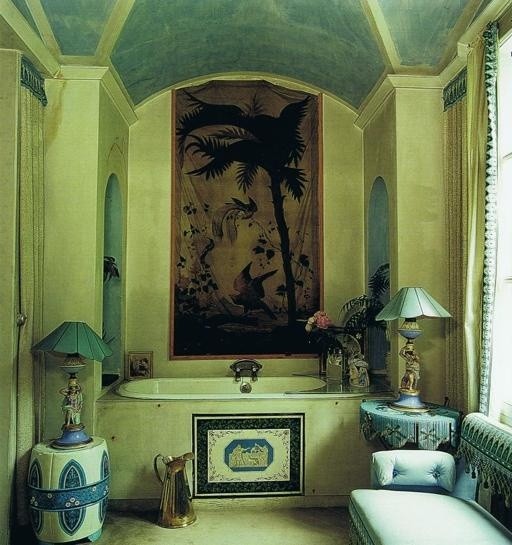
[349,414,512,545]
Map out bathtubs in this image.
[123,376,326,398]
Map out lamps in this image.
[36,321,110,447]
[375,288,452,412]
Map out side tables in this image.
[360,400,460,449]
